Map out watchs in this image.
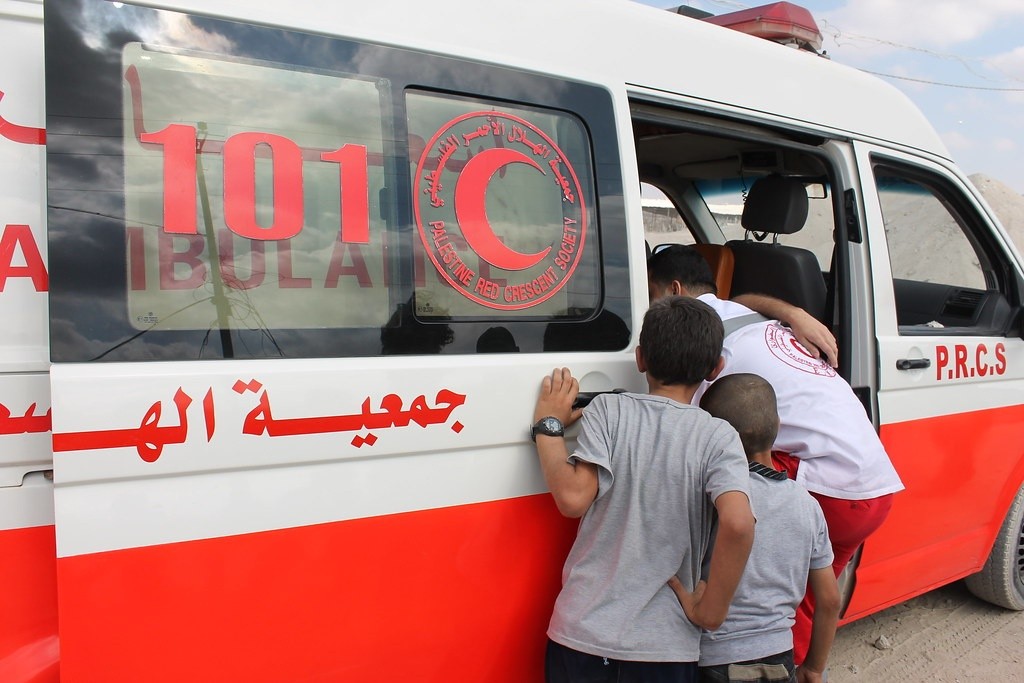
[530,416,564,444]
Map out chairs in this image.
[723,174,829,328]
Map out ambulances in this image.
[0,0,1024,683]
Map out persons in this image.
[645,239,838,368]
[698,373,841,683]
[648,244,905,683]
[530,295,757,683]
